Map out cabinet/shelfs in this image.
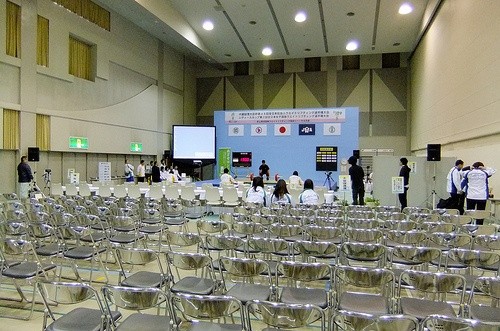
[99,162,111,181]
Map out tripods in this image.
[419,162,441,210]
[43,172,51,196]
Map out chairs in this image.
[0,182,500,331]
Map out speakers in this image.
[427,144,441,161]
[27,147,40,162]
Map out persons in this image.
[298,179,319,204]
[148,159,182,182]
[219,167,238,188]
[289,171,304,190]
[136,159,146,183]
[123,159,134,182]
[398,157,411,213]
[347,156,365,205]
[446,159,496,225]
[17,155,34,199]
[258,159,270,180]
[245,177,266,206]
[270,179,292,203]
